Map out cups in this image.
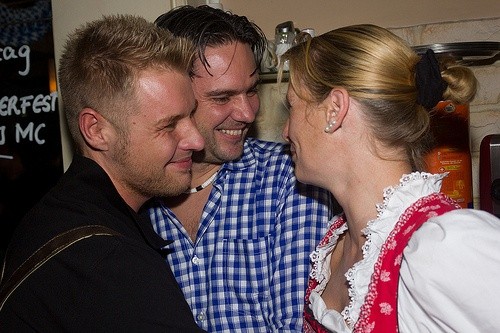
[274,21,300,71]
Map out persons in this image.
[143,5,333,333]
[0,13,206,333]
[277,24,500,333]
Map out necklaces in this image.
[183,170,219,193]
